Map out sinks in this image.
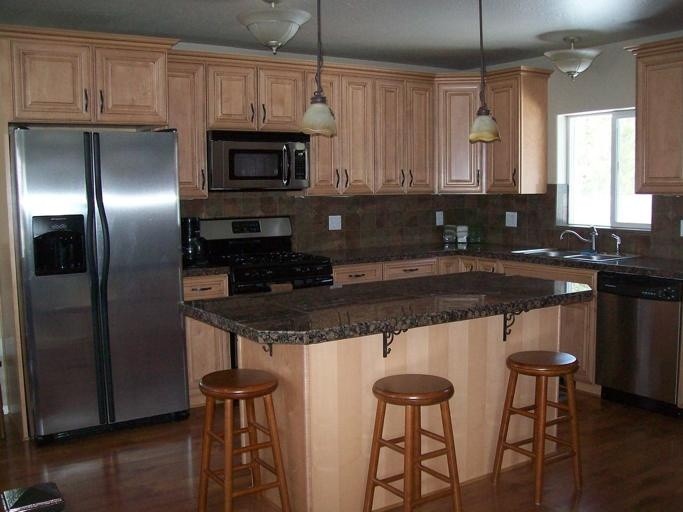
[563,254,621,262]
[511,248,593,261]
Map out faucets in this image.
[611,234,622,253]
[557,227,599,251]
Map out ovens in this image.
[228,274,332,294]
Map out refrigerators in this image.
[7,125,190,446]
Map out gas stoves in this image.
[218,251,330,271]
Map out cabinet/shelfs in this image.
[440,257,497,276]
[438,83,484,193]
[486,76,546,196]
[504,266,595,385]
[9,39,168,126]
[210,62,307,134]
[181,274,230,403]
[636,49,683,194]
[302,73,436,194]
[331,259,436,284]
[152,64,208,201]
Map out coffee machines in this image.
[181,217,213,268]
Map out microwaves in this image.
[206,132,312,194]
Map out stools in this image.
[362,372,464,511]
[196,368,291,511]
[492,350,584,506]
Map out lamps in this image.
[541,33,601,82]
[233,1,338,139]
[468,0,503,146]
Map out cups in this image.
[442,225,484,244]
